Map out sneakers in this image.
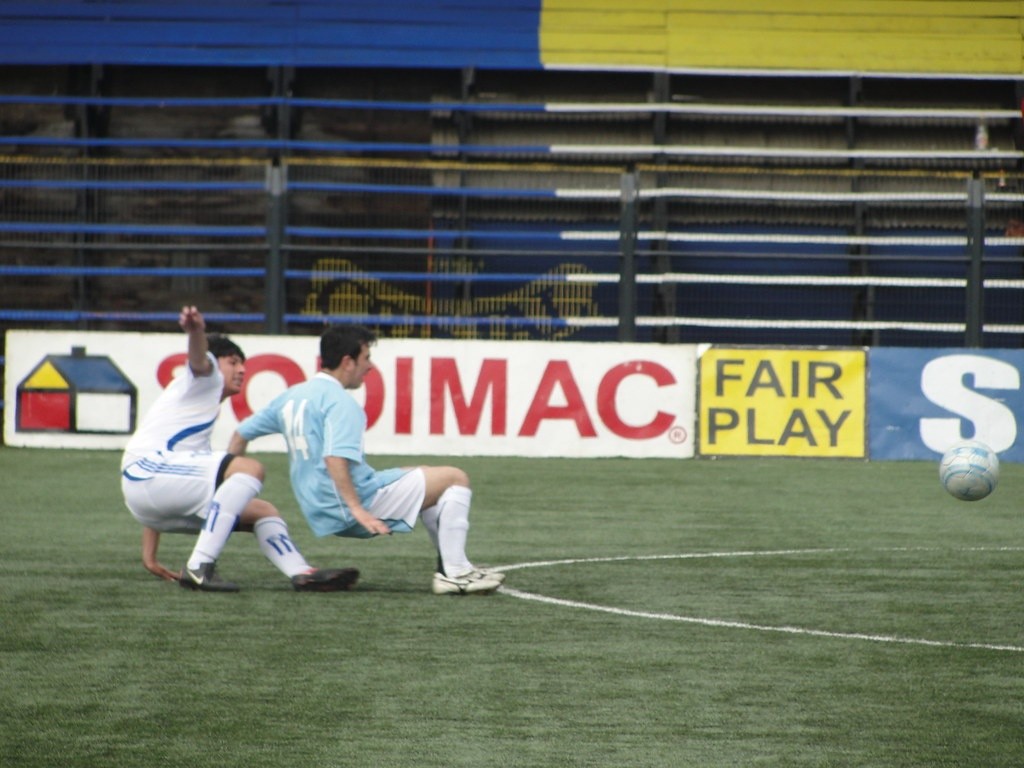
[432,569,501,595]
[469,569,506,582]
[179,561,240,593]
[292,566,360,592]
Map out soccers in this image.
[939,442,1001,501]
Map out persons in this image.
[975,129,1006,188]
[229,326,507,595]
[120,305,361,591]
